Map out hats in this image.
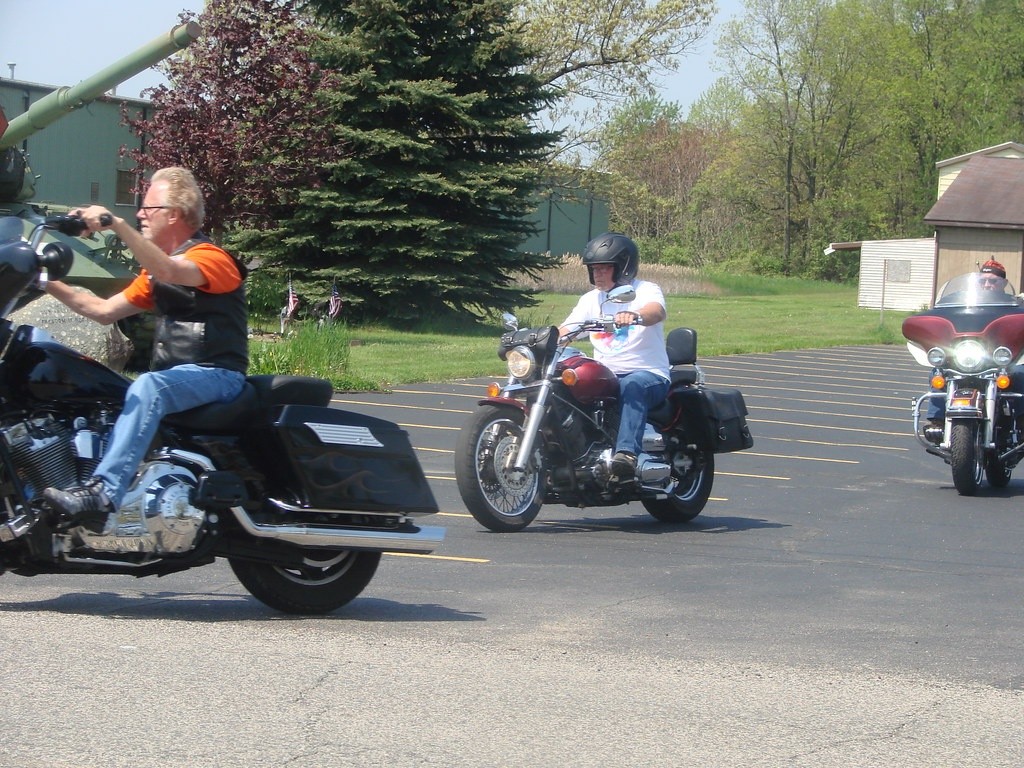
[981,260,1006,280]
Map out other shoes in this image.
[924,423,944,445]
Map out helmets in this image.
[582,234,639,285]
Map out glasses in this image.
[979,276,1004,284]
[137,202,175,212]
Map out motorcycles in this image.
[0,204,448,618]
[444,284,718,533]
[900,268,1024,494]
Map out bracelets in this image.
[38,273,48,290]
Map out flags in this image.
[329,283,341,318]
[287,285,300,315]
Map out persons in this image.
[923,260,1024,446]
[33,167,248,535]
[557,231,672,476]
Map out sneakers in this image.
[611,452,638,476]
[44,476,111,534]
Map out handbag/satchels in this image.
[684,384,754,454]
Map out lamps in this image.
[823,243,835,255]
